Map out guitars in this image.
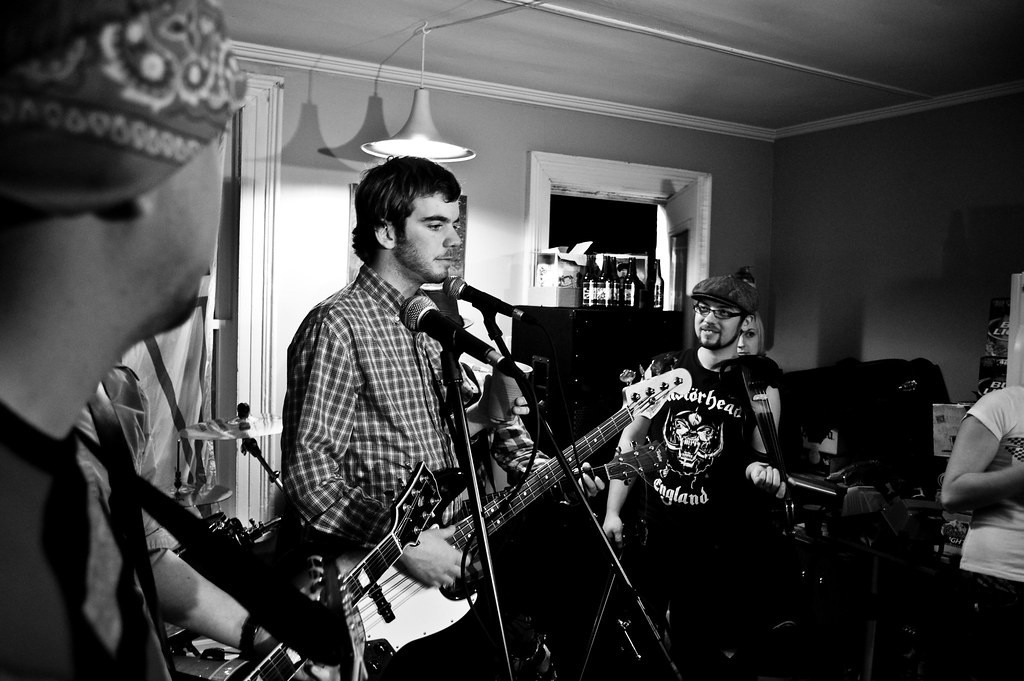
[240,460,443,680]
[285,366,694,681]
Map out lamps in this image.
[361,24,477,162]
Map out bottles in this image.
[647,259,664,311]
[622,258,643,307]
[581,253,621,308]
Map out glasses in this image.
[693,299,744,319]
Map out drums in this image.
[200,511,256,548]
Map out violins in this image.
[717,354,803,539]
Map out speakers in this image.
[509,306,685,512]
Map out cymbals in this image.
[159,482,235,508]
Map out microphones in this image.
[444,276,524,320]
[399,296,526,380]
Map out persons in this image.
[284,157,602,680]
[942,389,1024,681]
[603,275,788,681]
[0,0,362,681]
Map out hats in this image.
[691,275,759,315]
[0,0,247,208]
